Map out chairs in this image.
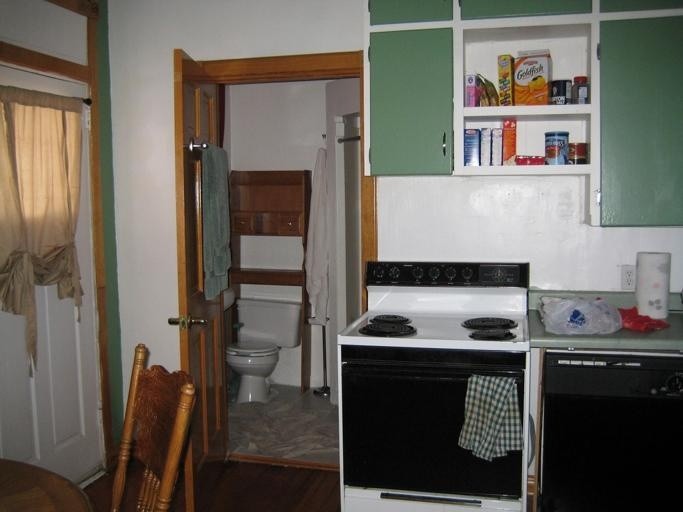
[98,335,201,510]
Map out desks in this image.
[0,452,95,511]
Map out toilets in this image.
[225,297,304,407]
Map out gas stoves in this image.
[337,308,529,350]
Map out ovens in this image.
[338,343,528,512]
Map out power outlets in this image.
[621,265,636,291]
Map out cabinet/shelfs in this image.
[456,0,594,178]
[360,1,455,179]
[579,2,681,230]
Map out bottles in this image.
[572,76,588,105]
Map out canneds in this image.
[516,131,588,166]
[551,79,573,105]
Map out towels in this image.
[301,146,336,329]
[454,371,527,466]
[196,137,236,306]
